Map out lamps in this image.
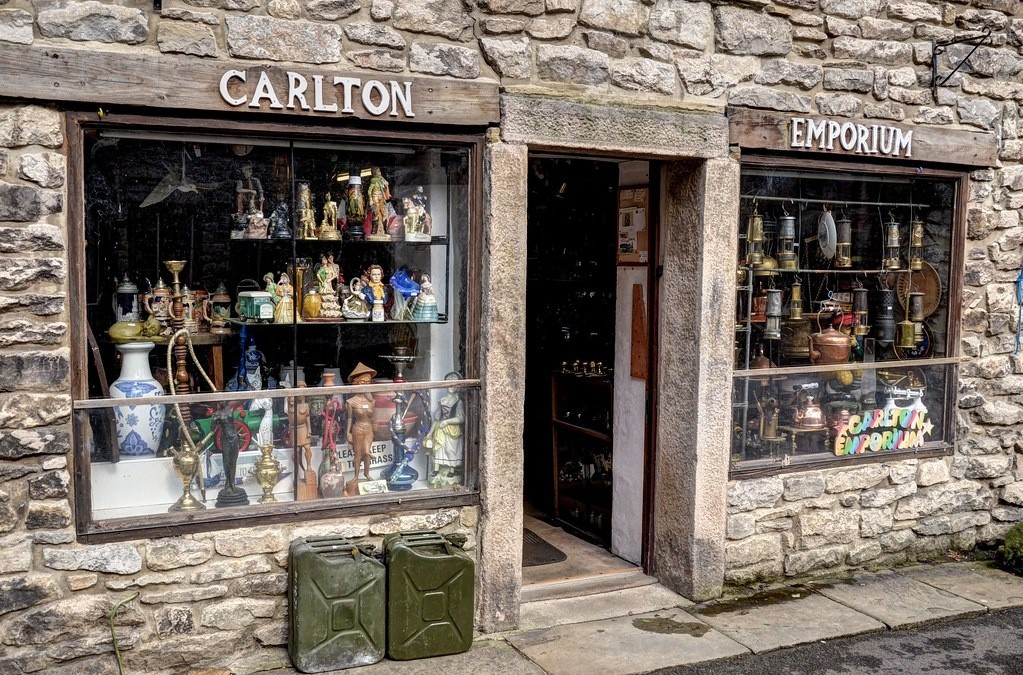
[737,186,927,359]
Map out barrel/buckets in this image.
[383,530,475,661]
[287,536,387,673]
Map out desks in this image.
[129,331,229,393]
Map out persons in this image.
[369,166,389,234]
[422,371,465,477]
[346,371,376,483]
[263,251,439,324]
[235,165,265,219]
[323,192,339,229]
[210,399,247,493]
[288,380,313,472]
[412,185,428,224]
[300,201,316,238]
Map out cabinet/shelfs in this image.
[229,234,451,451]
[550,369,615,552]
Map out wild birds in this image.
[138,172,222,209]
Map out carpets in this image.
[521,526,568,568]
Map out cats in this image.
[246,366,294,450]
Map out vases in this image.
[110,342,166,459]
